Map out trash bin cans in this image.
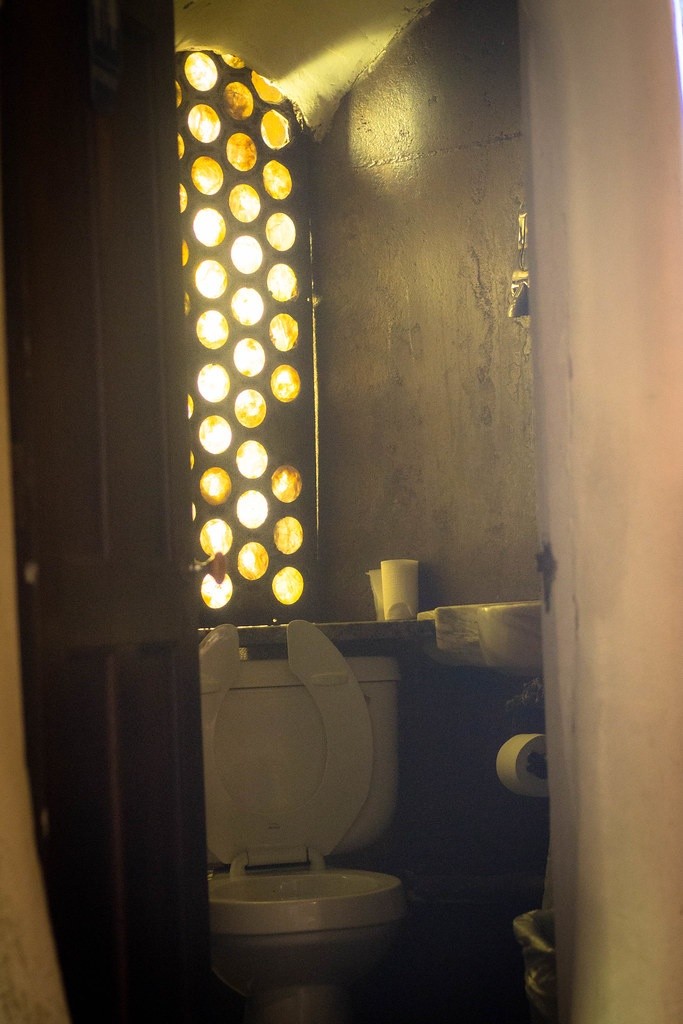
[514,906,556,1024]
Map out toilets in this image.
[195,617,410,1024]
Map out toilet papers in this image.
[368,558,419,622]
[496,733,550,797]
